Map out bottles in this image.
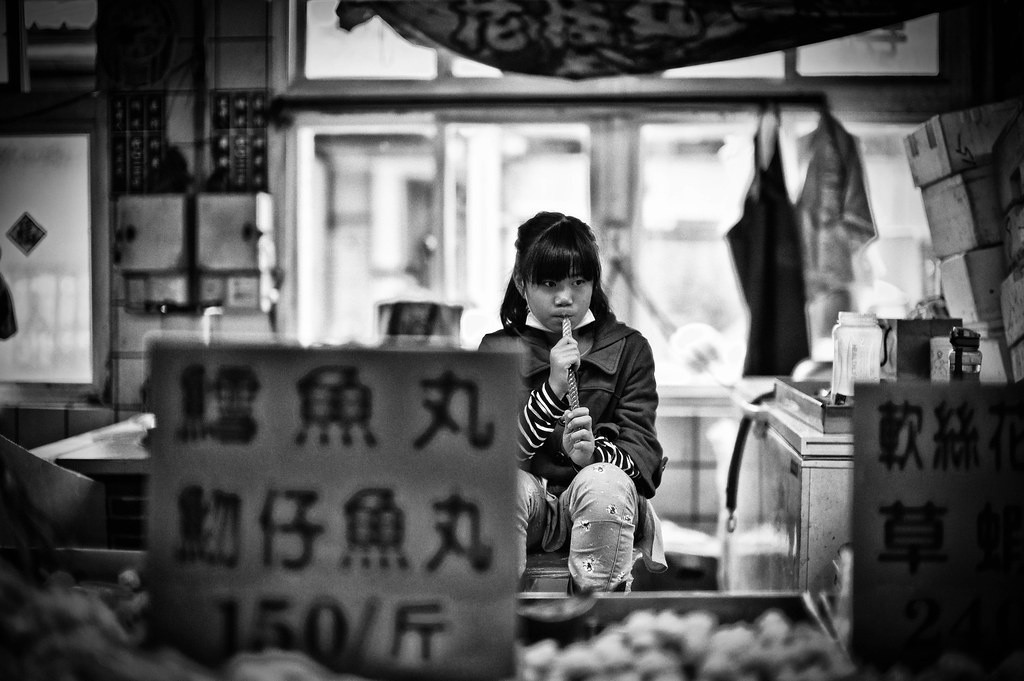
[831,311,883,405]
[948,326,983,381]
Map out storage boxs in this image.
[112,195,287,277]
[50,545,844,680]
[903,99,1023,385]
[774,378,852,436]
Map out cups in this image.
[930,336,953,380]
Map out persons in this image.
[479,211,668,593]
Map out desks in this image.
[54,432,156,553]
